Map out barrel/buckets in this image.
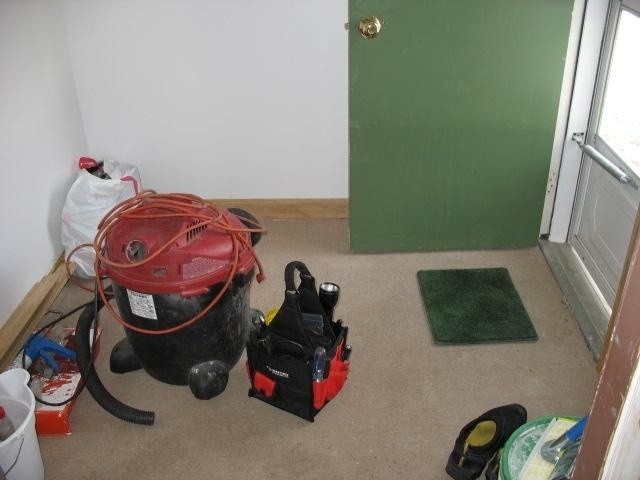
[0,368,46,480]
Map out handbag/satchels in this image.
[245,261,350,423]
[61,159,144,281]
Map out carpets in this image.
[417,266,539,345]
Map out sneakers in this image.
[445,403,527,480]
[485,444,503,480]
